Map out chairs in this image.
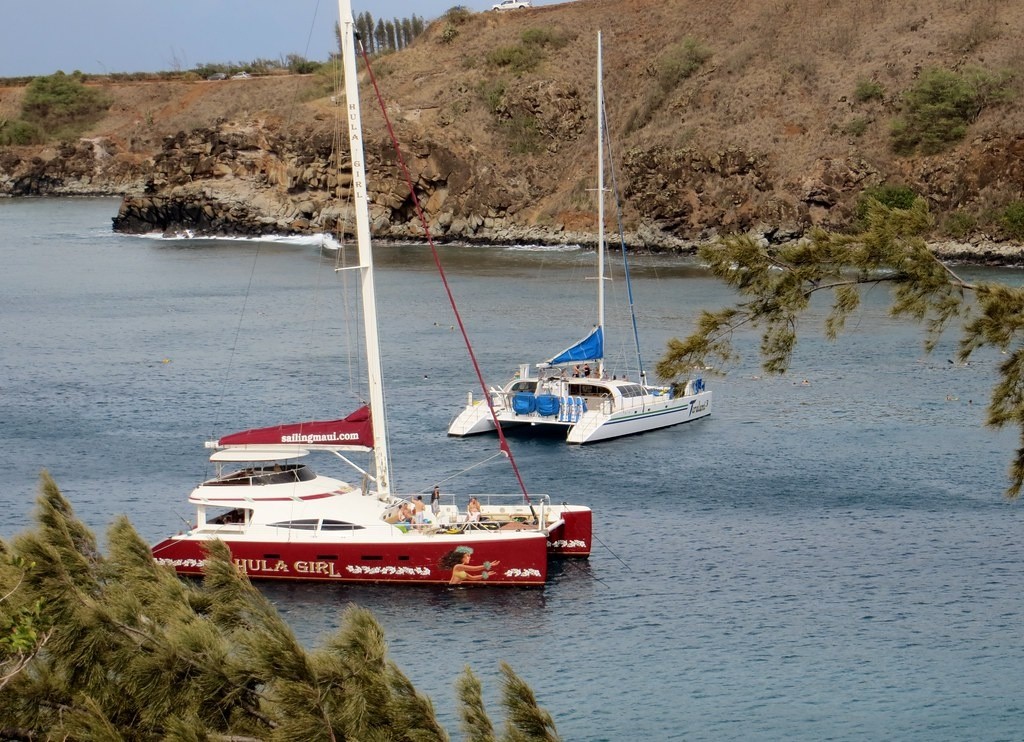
[395,515,490,527]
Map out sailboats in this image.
[440,26,715,448]
[144,2,593,590]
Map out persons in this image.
[582,364,592,378]
[430,486,439,517]
[397,505,414,530]
[466,497,480,526]
[403,503,411,515]
[572,365,581,377]
[436,545,500,585]
[411,493,425,533]
